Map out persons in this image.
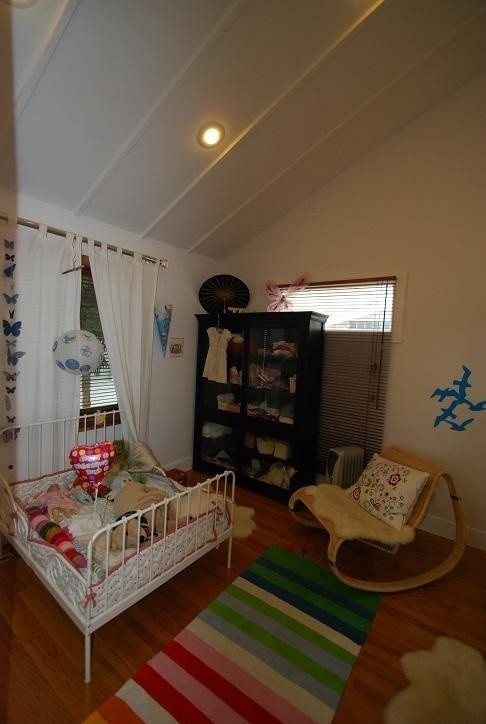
[42,482,76,523]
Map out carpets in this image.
[81,543,385,724]
[233,504,257,539]
[381,636,485,724]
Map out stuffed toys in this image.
[110,479,177,552]
[27,505,86,569]
[108,470,133,500]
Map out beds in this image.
[0,410,236,683]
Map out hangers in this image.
[213,314,226,334]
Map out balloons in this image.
[69,440,115,486]
[49,330,108,375]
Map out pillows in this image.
[344,453,432,532]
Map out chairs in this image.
[288,444,467,594]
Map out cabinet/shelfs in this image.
[193,312,328,512]
